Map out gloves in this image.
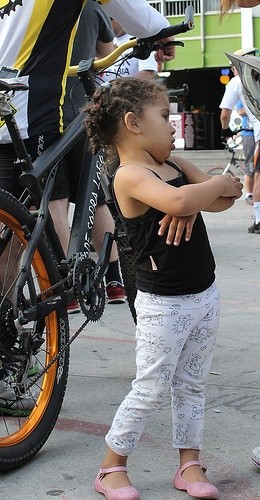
[221,128,233,139]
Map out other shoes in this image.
[251,447,260,468]
[94,466,139,500]
[174,461,218,498]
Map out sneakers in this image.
[66,299,81,313]
[106,283,127,304]
[245,195,253,205]
[248,221,260,234]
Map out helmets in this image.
[228,47,259,67]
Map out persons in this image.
[93,16,138,90]
[48,0,125,314]
[82,77,244,500]
[0,0,177,417]
[220,48,260,234]
[236,98,256,207]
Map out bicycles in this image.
[205,126,255,180]
[0,21,194,474]
